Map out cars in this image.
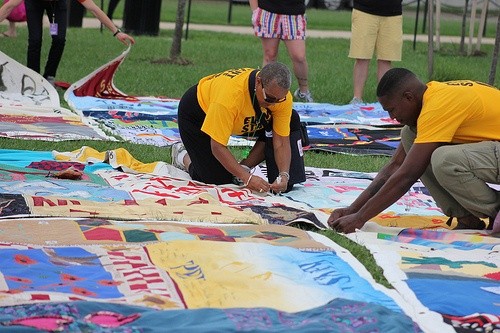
[229,0,353,10]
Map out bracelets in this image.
[279,172,289,180]
[245,174,252,185]
[113,29,121,37]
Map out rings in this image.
[260,189,263,192]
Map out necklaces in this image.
[247,92,265,150]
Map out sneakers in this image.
[293,87,313,102]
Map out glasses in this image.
[261,79,286,102]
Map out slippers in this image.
[0,31,17,37]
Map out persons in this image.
[0,0,135,88]
[249,0,313,102]
[348,0,403,104]
[327,67,500,234]
[172,63,306,193]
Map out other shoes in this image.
[171,141,187,170]
[348,96,363,104]
[486,216,494,229]
[232,162,252,185]
[452,219,486,230]
